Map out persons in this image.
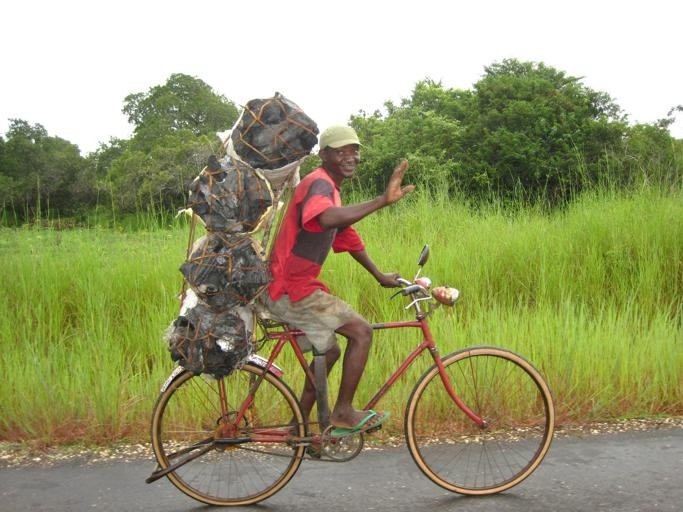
[256,126,415,438]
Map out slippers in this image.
[326,407,392,440]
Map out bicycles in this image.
[147,245,554,507]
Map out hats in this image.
[317,123,365,152]
